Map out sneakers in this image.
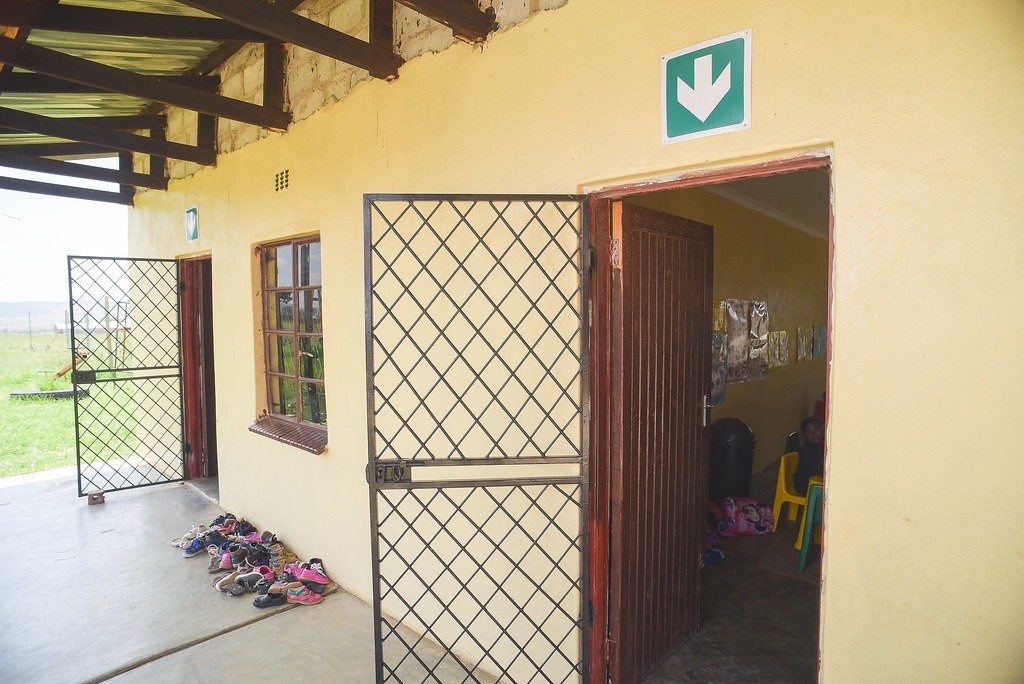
[252,592,285,608]
[287,586,323,604]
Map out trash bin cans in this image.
[711,417,757,499]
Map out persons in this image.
[793,418,825,498]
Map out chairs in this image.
[772,430,823,573]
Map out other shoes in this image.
[171,511,329,597]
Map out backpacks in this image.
[718,496,774,538]
